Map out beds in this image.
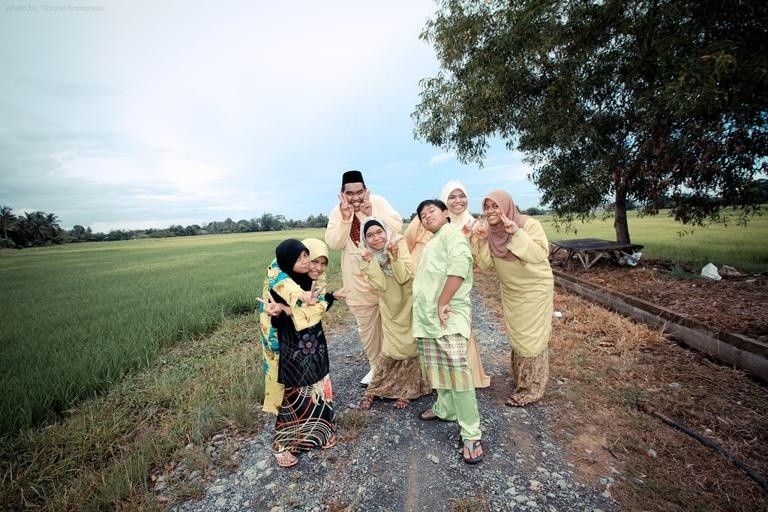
[549,238,644,273]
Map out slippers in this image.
[275,450,298,467]
[419,410,437,421]
[463,438,484,465]
[506,398,529,407]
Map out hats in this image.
[343,170,364,185]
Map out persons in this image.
[261,237,329,416]
[355,215,432,411]
[401,179,491,389]
[412,200,491,464]
[472,190,555,407]
[256,238,351,467]
[324,171,403,388]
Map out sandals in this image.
[394,398,412,409]
[360,394,374,410]
[322,433,337,449]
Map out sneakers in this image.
[360,368,374,386]
